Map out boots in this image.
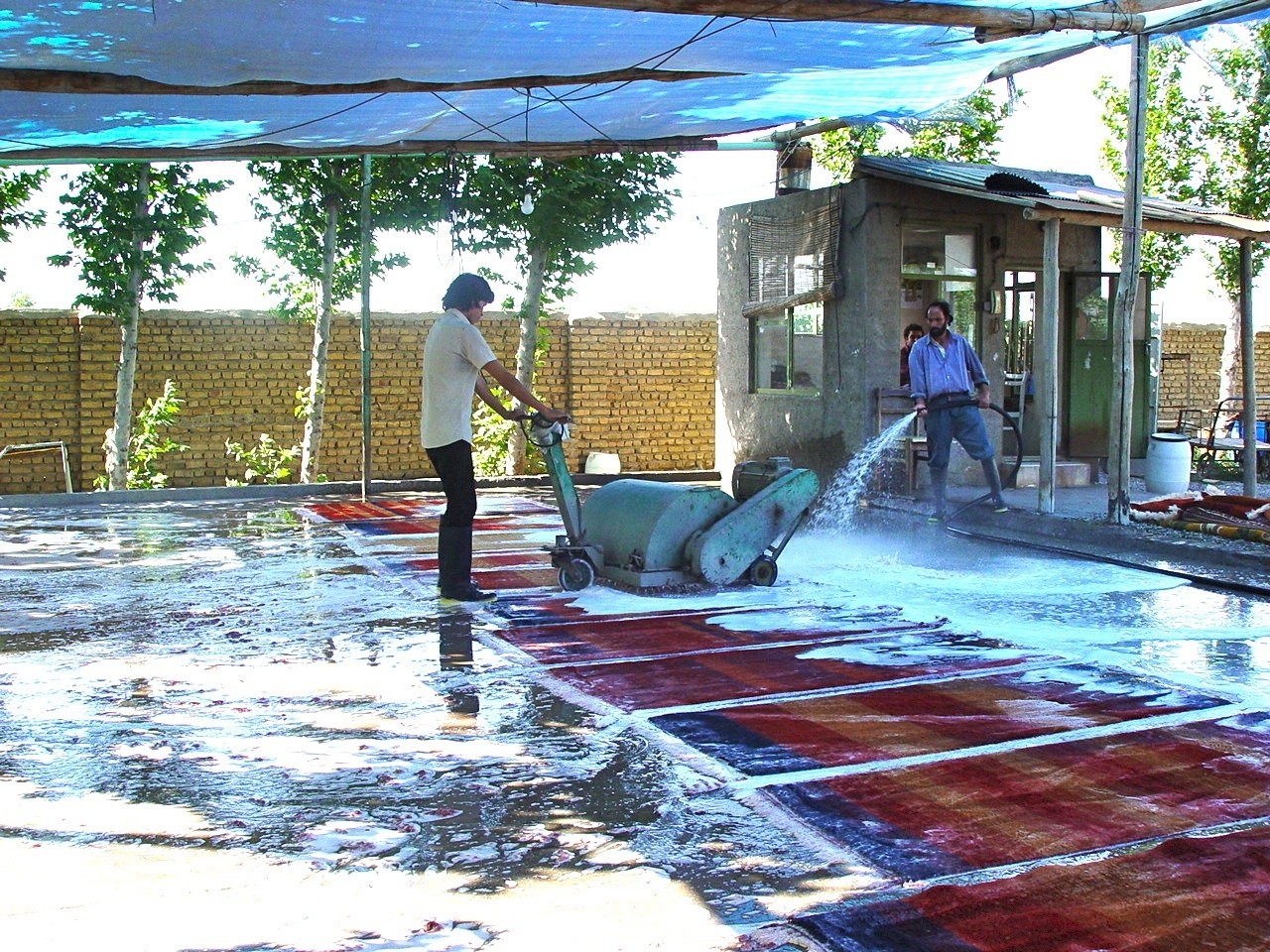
[928,467,945,522]
[980,456,1009,513]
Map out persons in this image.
[421,273,571,608]
[899,300,1008,522]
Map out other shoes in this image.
[440,587,497,605]
[437,579,479,591]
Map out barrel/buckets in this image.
[1145,433,1192,494]
[1231,421,1267,456]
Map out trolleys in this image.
[515,403,820,592]
[1177,395,1270,477]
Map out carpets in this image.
[291,491,1270,952]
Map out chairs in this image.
[794,371,816,388]
[770,365,793,389]
[1003,371,1029,456]
[874,387,932,498]
